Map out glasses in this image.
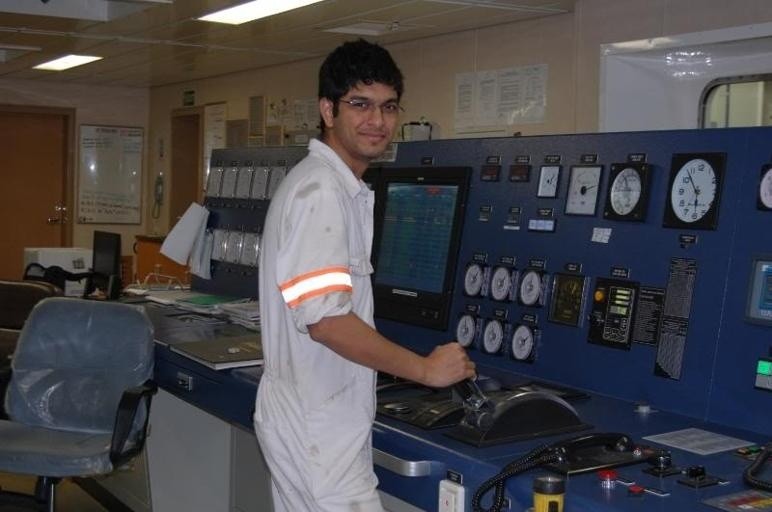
[338,98,407,114]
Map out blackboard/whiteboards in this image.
[77,123,144,224]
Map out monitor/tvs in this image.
[91,231,122,274]
[368,177,463,310]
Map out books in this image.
[144,289,262,332]
[168,334,264,371]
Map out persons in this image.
[245,36,479,511]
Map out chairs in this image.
[0,297,157,511]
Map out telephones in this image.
[154,177,164,202]
[546,432,633,464]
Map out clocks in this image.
[661,151,728,230]
